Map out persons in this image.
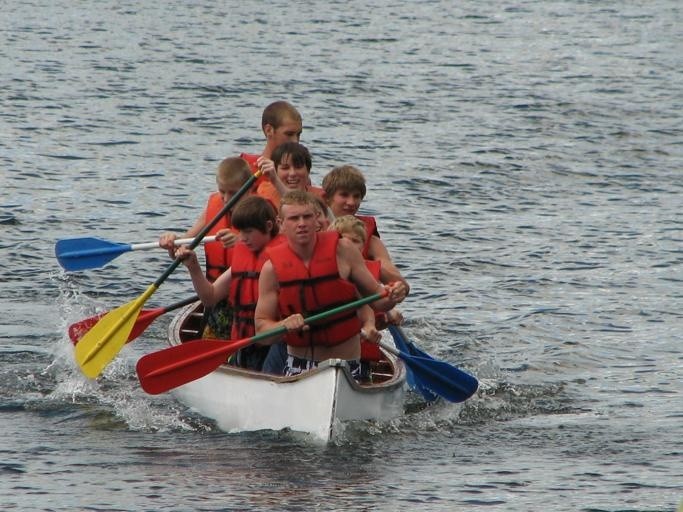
[260,213,382,376]
[158,157,291,366]
[321,165,409,295]
[252,190,408,385]
[239,100,301,186]
[266,141,323,208]
[173,195,279,371]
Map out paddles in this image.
[361,311,479,402]
[73,166,267,377]
[137,290,391,395]
[54,235,223,270]
[69,295,202,347]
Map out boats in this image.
[168,290,412,445]
